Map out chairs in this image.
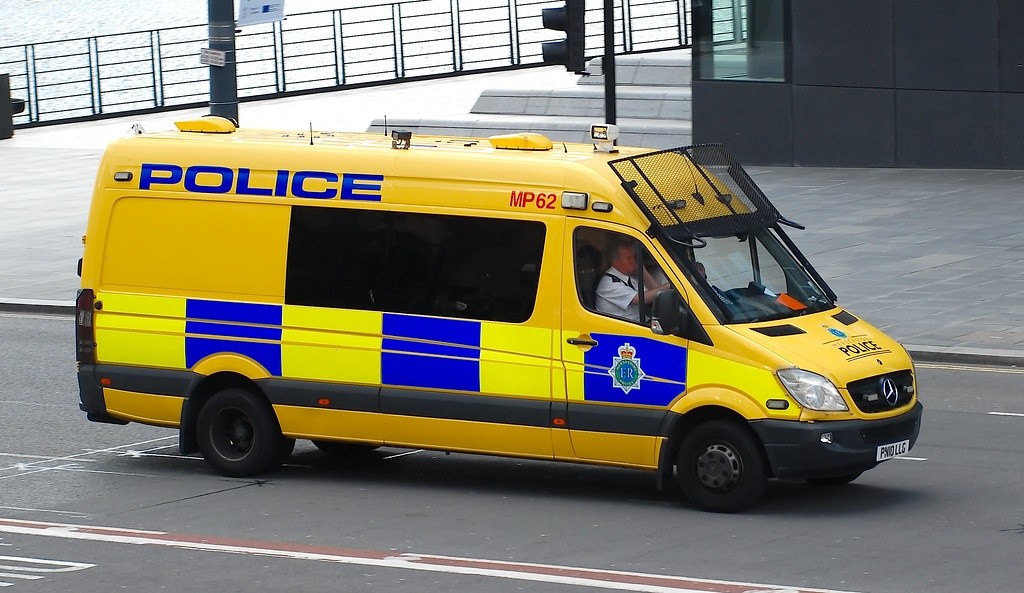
[577,244,604,310]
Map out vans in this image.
[73,115,924,515]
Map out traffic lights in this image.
[541,0,584,72]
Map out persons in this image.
[595,245,706,322]
